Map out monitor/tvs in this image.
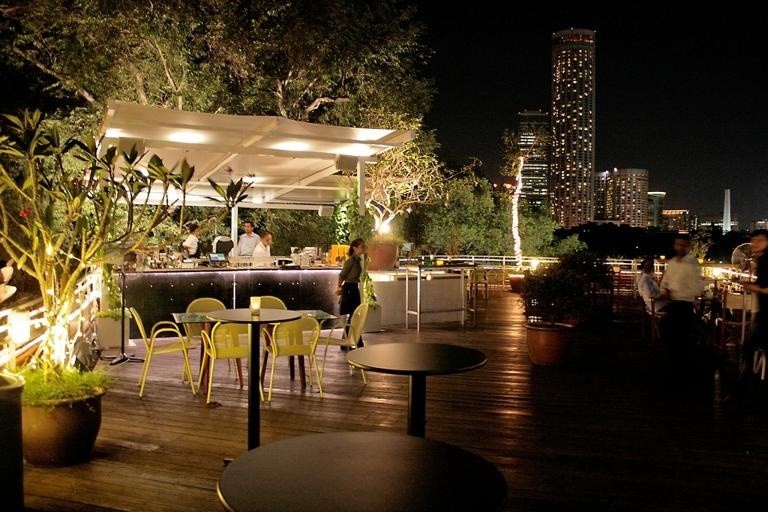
[209,253,226,263]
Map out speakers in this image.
[117,137,144,156]
[336,154,358,171]
[318,206,334,216]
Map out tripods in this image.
[102,272,145,365]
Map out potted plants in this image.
[1,104,256,470]
[515,270,578,365]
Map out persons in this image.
[334,238,367,351]
[660,233,703,323]
[180,224,201,260]
[636,257,671,319]
[718,227,768,408]
[252,231,273,257]
[229,221,261,257]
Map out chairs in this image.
[601,259,767,377]
[124,296,374,406]
